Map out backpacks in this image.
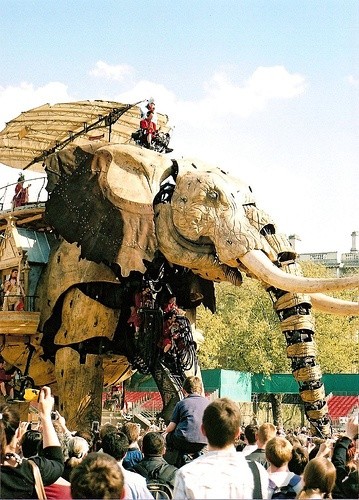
[265,475,302,499]
[135,465,177,499]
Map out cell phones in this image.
[23,388,40,403]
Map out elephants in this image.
[1,143,358,440]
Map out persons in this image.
[0,376,359,500]
[13,177,31,207]
[129,287,151,338]
[161,293,186,362]
[3,269,26,310]
[140,111,173,153]
[0,359,21,400]
